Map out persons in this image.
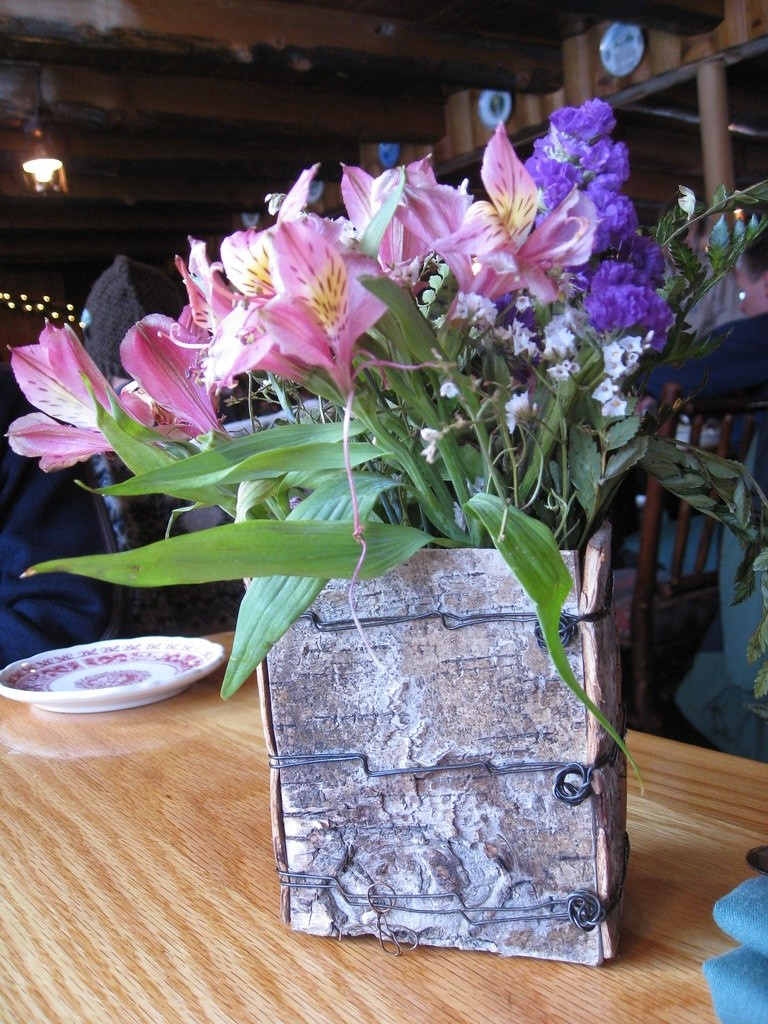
[578,226,768,549]
[0,256,284,671]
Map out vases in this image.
[244,518,628,967]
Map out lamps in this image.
[22,65,64,186]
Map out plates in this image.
[0,635,226,713]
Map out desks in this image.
[0,629,768,1023]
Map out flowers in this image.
[5,98,768,797]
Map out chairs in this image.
[615,376,768,726]
